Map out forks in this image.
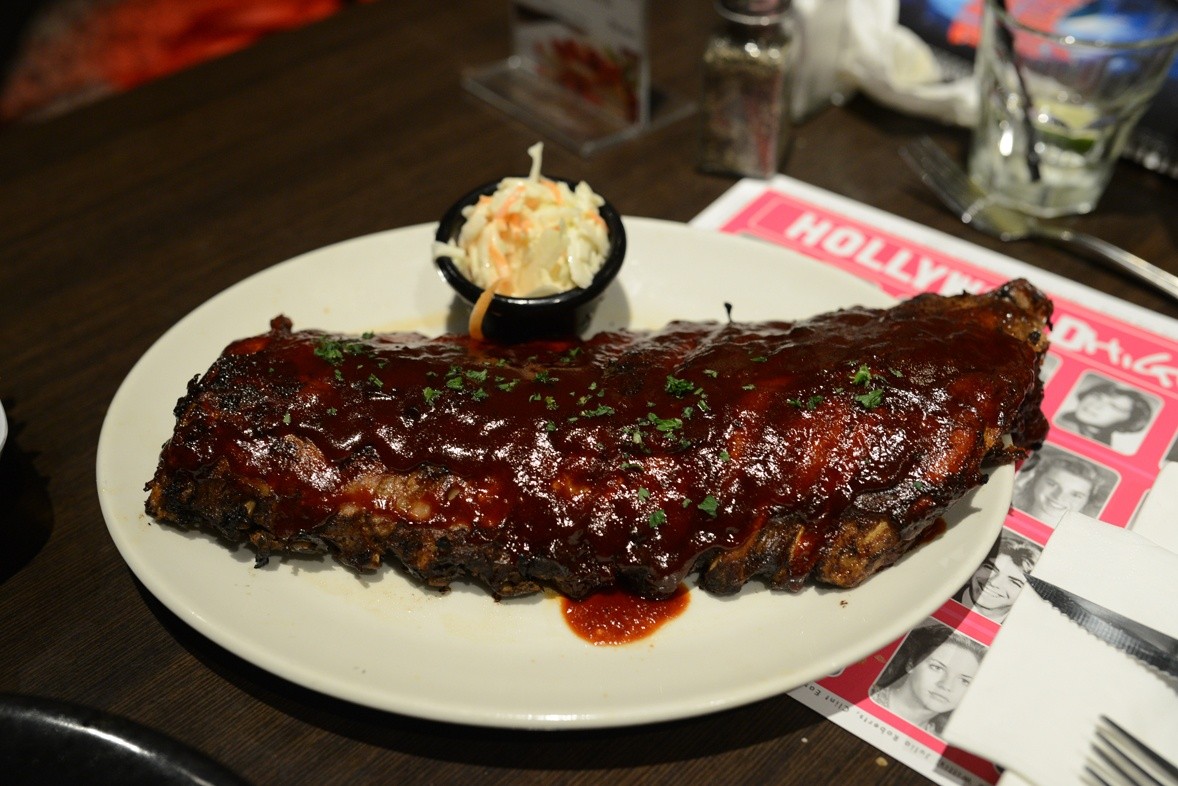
[887,137,1178,306]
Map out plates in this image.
[95,217,1014,727]
[0,690,251,785]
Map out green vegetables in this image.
[281,331,888,528]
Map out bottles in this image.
[695,1,805,181]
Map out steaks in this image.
[142,274,1054,597]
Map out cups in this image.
[967,0,1178,221]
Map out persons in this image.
[951,535,1044,625]
[1012,455,1099,530]
[1054,381,1153,447]
[869,623,989,740]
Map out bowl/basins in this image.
[437,175,628,319]
